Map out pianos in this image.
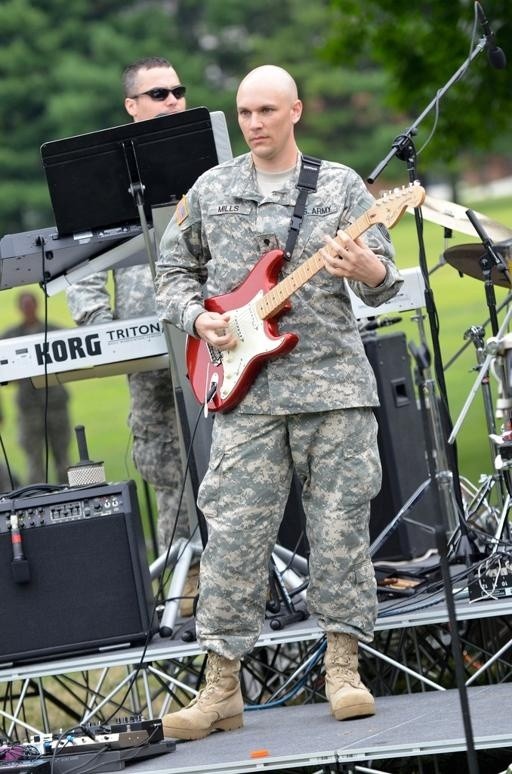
[2,226,155,290]
[0,266,430,383]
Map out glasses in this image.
[131,86,188,102]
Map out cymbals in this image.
[379,191,512,243]
[443,242,511,288]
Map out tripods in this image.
[361,345,493,559]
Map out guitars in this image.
[184,180,425,412]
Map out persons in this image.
[67,57,200,616]
[154,66,404,745]
[0,294,70,484]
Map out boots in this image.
[160,653,246,742]
[179,561,200,616]
[322,635,379,723]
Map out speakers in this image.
[1,480,161,670]
[276,333,442,562]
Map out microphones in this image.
[474,11,506,70]
[9,507,32,584]
